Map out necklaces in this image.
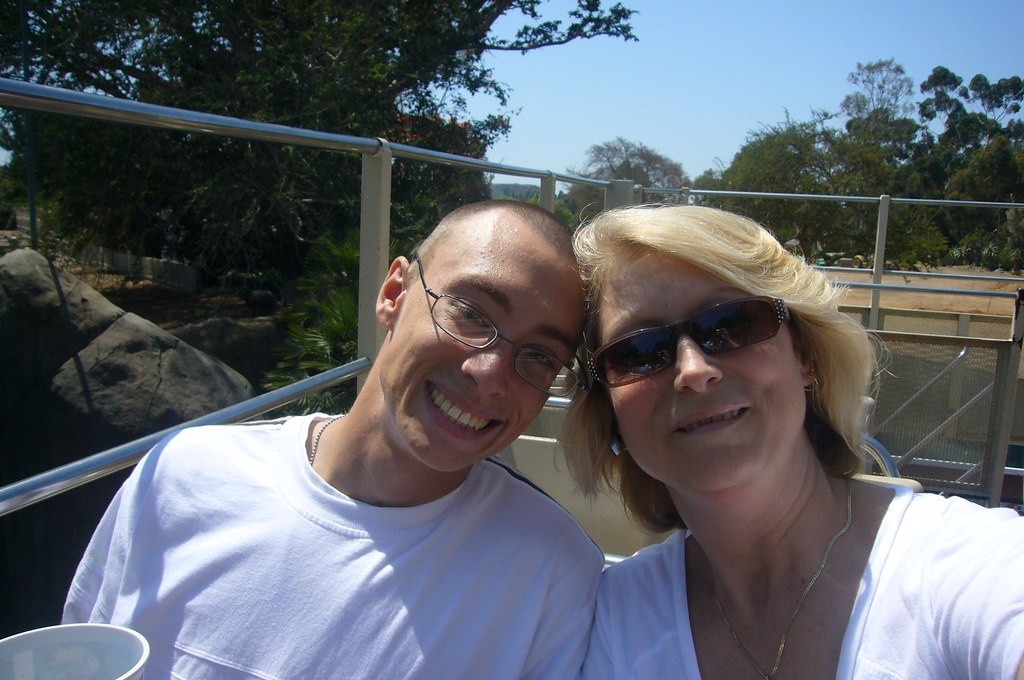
[310,414,346,468]
[709,471,852,680]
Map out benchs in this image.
[498,399,921,568]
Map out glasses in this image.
[587,295,789,388]
[413,249,582,397]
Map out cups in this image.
[0,621,150,680]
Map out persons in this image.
[61,199,607,680]
[559,203,1024,680]
